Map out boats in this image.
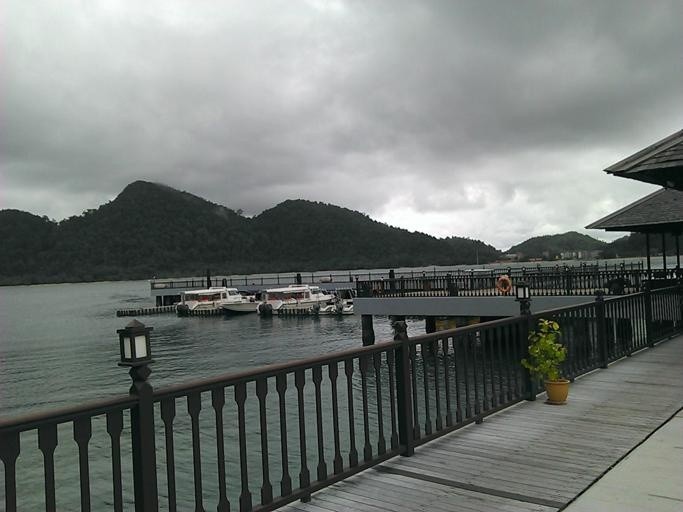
[175,286,354,316]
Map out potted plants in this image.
[520,317,572,408]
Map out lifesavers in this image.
[497,277,511,292]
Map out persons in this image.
[255,291,262,300]
[250,297,254,302]
